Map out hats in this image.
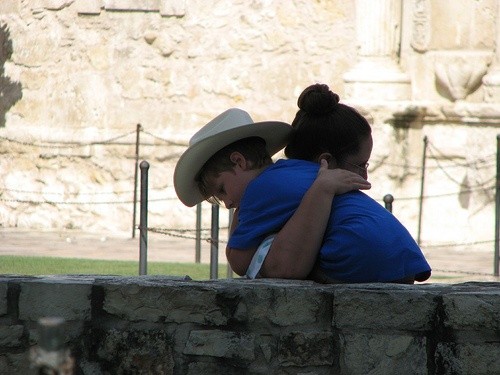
[173,108,293,208]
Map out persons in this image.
[173,108,432,284]
[241,83,373,279]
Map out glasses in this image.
[340,153,369,172]
